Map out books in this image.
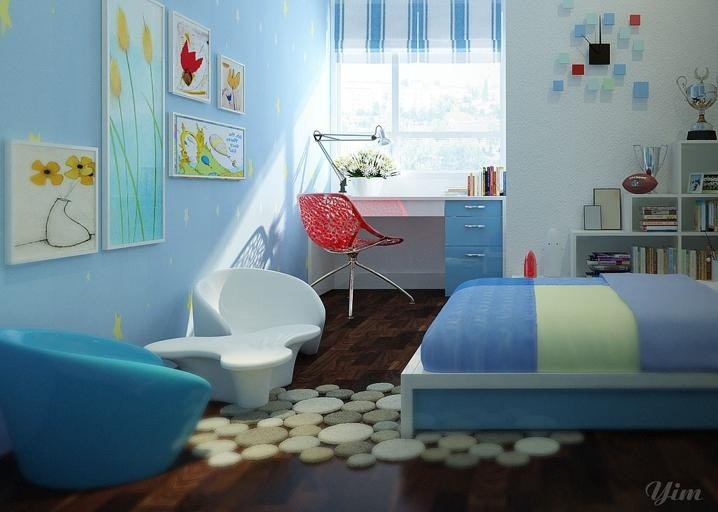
[584,199,718,282]
[467,165,506,197]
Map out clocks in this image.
[554,2,650,99]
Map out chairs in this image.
[296,193,416,319]
[0,327,211,491]
[192,268,326,355]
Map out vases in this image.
[43,196,89,247]
[346,176,390,196]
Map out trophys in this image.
[632,145,668,193]
[677,67,718,140]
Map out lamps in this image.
[312,125,391,193]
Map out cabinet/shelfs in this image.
[569,139,718,278]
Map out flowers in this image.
[24,151,94,200]
[333,151,402,181]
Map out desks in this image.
[300,195,506,294]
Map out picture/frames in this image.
[5,138,99,266]
[216,54,246,115]
[169,11,211,103]
[169,113,246,180]
[99,0,168,251]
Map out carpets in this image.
[188,383,587,468]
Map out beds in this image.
[400,274,716,438]
